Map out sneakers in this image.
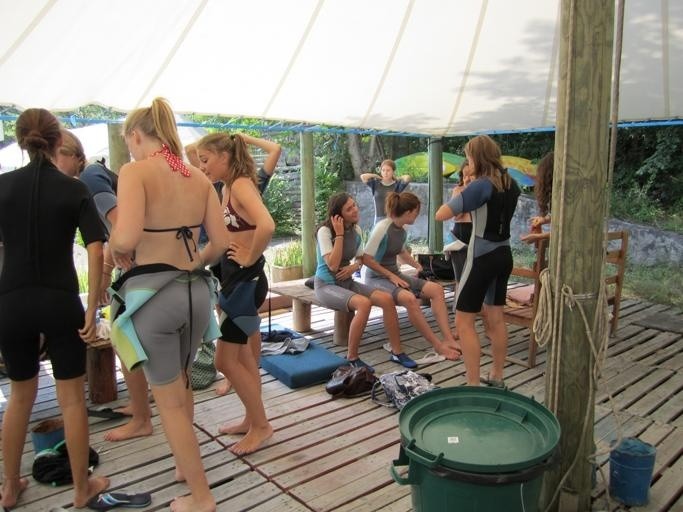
[349,359,374,373]
[390,350,416,368]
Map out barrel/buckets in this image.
[609,438,658,507]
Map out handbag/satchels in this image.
[418,253,454,281]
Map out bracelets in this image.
[333,233,345,240]
[354,261,362,270]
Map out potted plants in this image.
[271,238,302,283]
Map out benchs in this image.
[268,257,457,347]
[0,313,119,404]
[473,228,628,368]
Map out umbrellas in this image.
[0,0,682,147]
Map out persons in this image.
[106,99,232,510]
[0,106,110,511]
[180,131,282,398]
[432,136,521,387]
[312,192,419,377]
[519,150,555,246]
[450,159,476,252]
[50,129,154,443]
[359,191,463,363]
[360,159,410,227]
[195,129,274,456]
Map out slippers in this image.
[87,407,122,419]
[383,343,391,352]
[416,352,446,365]
[88,492,151,510]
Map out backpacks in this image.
[326,365,378,400]
[371,370,439,410]
